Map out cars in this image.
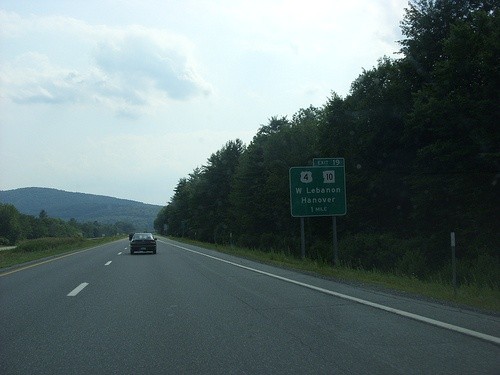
[129,232,158,254]
[129,232,134,240]
